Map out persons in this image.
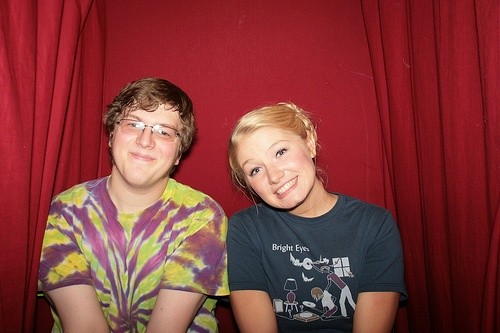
[34,77,230,333]
[225,102,409,333]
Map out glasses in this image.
[108,119,186,150]
